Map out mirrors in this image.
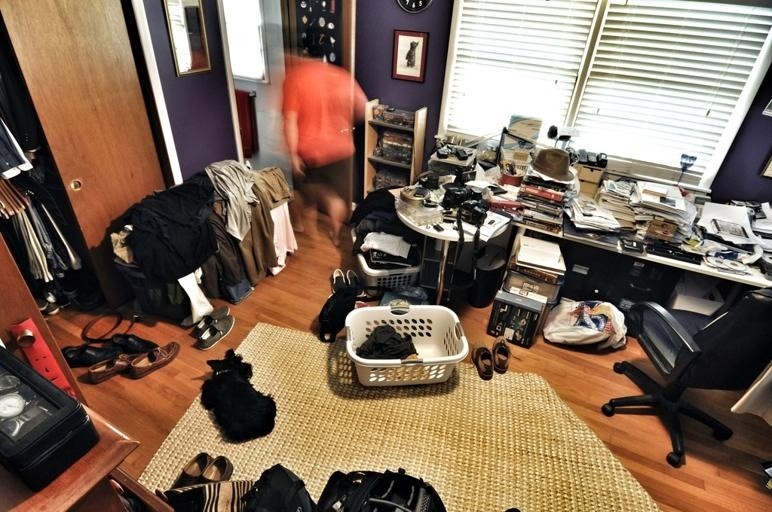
[160,0,211,78]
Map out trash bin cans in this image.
[468,244,507,308]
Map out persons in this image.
[263,31,372,248]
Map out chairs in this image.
[603,290,772,469]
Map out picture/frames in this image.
[391,30,427,83]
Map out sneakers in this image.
[330,268,365,298]
[194,305,235,350]
[170,452,233,489]
[471,336,511,380]
[34,280,99,315]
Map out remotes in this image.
[579,150,598,163]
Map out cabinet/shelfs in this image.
[0,401,143,511]
[363,99,428,203]
[493,196,769,326]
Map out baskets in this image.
[345,305,469,386]
[350,228,423,296]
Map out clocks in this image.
[397,1,433,14]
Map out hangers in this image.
[0,171,31,223]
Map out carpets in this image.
[133,322,664,512]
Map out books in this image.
[509,162,698,284]
[711,219,749,238]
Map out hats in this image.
[530,149,575,181]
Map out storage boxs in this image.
[1,348,104,494]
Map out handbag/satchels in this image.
[318,287,356,334]
[241,464,446,512]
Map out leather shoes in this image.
[61,333,180,384]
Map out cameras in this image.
[442,166,489,227]
